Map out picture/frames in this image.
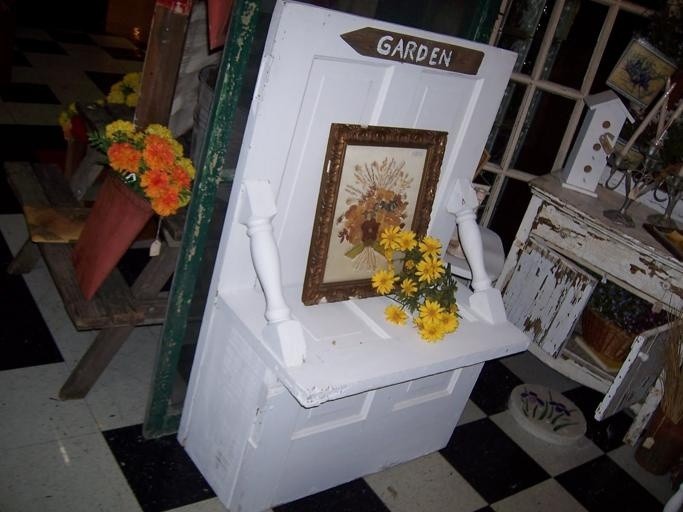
[301,123,450,307]
[608,36,676,107]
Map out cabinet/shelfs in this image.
[178,8,528,512]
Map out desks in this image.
[496,170,683,446]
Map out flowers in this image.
[517,385,584,435]
[70,124,194,296]
[337,159,411,274]
[63,74,144,203]
[371,228,464,342]
[620,52,664,97]
